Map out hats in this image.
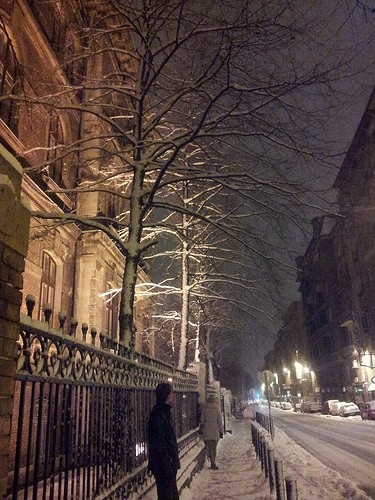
[207,395,216,402]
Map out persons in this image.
[199,395,223,469]
[148,383,181,500]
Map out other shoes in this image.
[211,463,214,469]
[212,464,218,470]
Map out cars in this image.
[294,401,319,412]
[262,400,292,410]
[360,400,375,420]
[331,401,360,417]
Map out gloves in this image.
[220,433,223,439]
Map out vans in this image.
[321,400,339,414]
